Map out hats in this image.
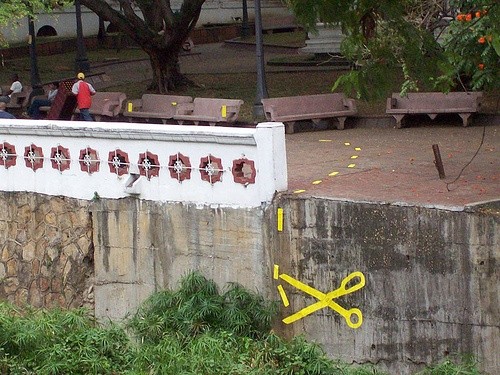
[78,73,84,79]
[43,77,60,85]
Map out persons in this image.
[27,81,62,120]
[71,72,97,122]
[0,73,22,104]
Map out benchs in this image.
[386,92,482,127]
[261,15,301,34]
[174,97,244,127]
[0,86,126,122]
[261,93,358,134]
[122,93,192,124]
